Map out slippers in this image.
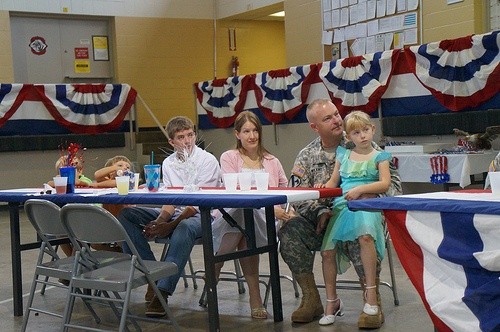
[203,262,222,285]
[251,307,267,319]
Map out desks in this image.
[393,150,500,192]
[0,187,344,332]
[348,189,500,332]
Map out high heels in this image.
[318,297,344,325]
[363,286,378,316]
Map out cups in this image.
[488,172,500,200]
[238,173,252,193]
[223,174,238,193]
[115,176,130,195]
[133,174,140,190]
[53,177,68,194]
[254,173,270,193]
[144,165,160,193]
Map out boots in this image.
[358,272,384,329]
[291,272,325,322]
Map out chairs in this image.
[24,199,399,332]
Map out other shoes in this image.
[58,278,70,285]
[144,286,155,302]
[146,291,169,315]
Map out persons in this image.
[117,116,221,317]
[201,110,295,320]
[313,110,393,326]
[47,156,93,287]
[86,155,134,251]
[277,98,404,329]
